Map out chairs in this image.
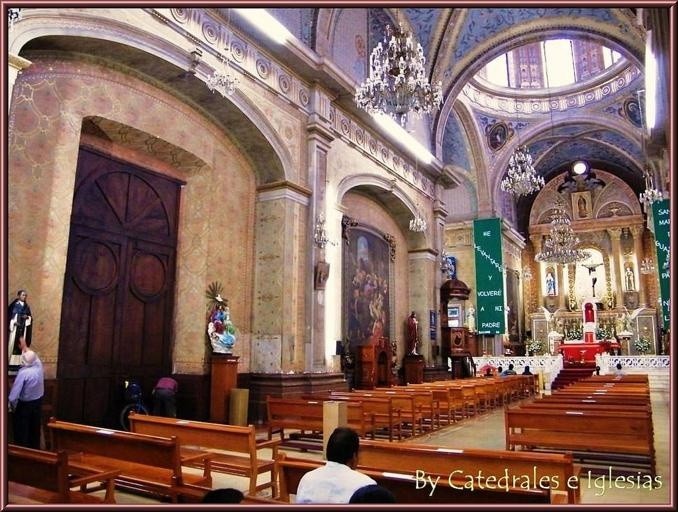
[265,372,498,445]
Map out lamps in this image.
[498,143,545,196]
[204,47,239,95]
[639,151,663,210]
[408,156,425,233]
[353,7,443,128]
[312,212,339,249]
[535,201,589,264]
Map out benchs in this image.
[130,410,278,501]
[47,416,213,502]
[273,446,570,504]
[499,373,656,455]
[328,435,585,503]
[4,442,123,505]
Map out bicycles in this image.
[120,381,149,433]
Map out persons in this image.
[584,263,601,288]
[199,489,244,504]
[625,267,634,291]
[613,364,624,375]
[151,376,179,419]
[8,289,34,371]
[592,366,605,376]
[407,311,419,355]
[297,427,376,505]
[8,337,44,450]
[546,273,554,295]
[483,364,534,394]
